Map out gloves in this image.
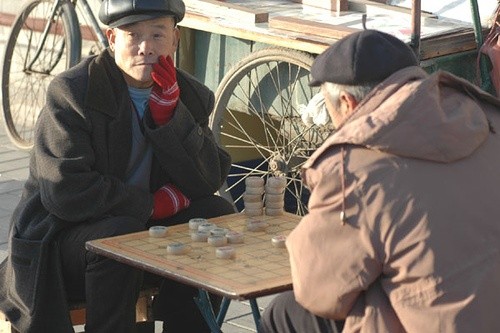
[151,182,191,220]
[150,55,181,125]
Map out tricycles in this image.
[0,0,494,224]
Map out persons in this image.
[259,29,500,333]
[0,0,238,333]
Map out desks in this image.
[85,211,303,333]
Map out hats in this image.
[307,14,420,88]
[98,0,186,29]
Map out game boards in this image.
[84,205,309,302]
